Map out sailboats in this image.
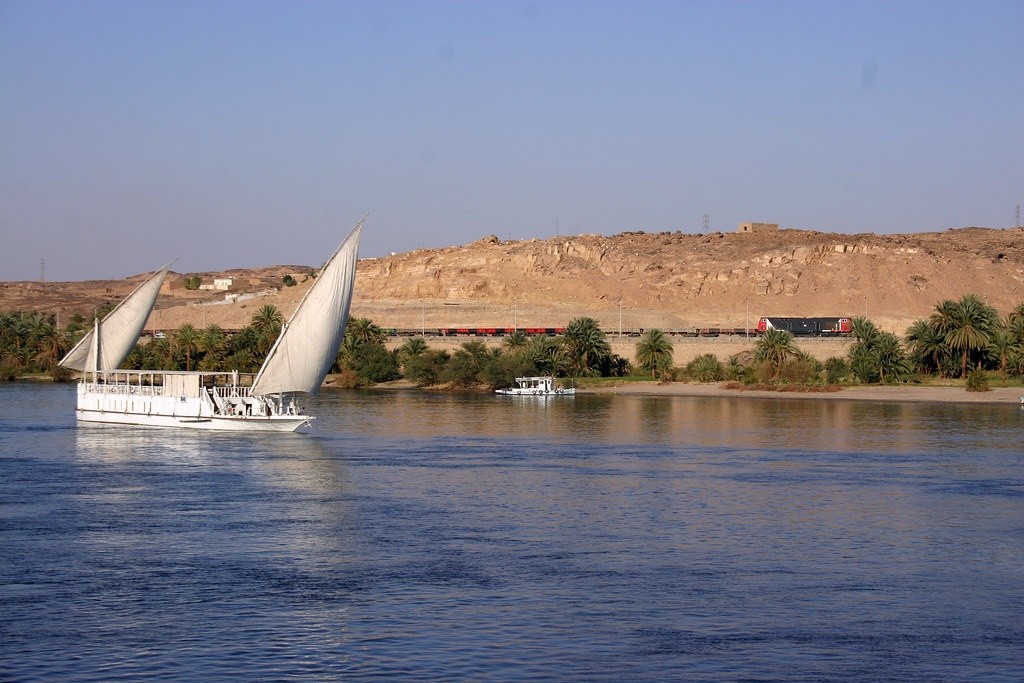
[58,216,365,433]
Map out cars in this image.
[155,333,165,338]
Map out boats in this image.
[495,376,576,395]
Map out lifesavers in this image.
[539,390,543,395]
[517,391,521,395]
[544,389,549,393]
[559,389,564,394]
[554,389,559,393]
[532,391,536,394]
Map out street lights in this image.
[745,297,748,337]
[864,297,868,320]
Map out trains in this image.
[757,317,854,337]
